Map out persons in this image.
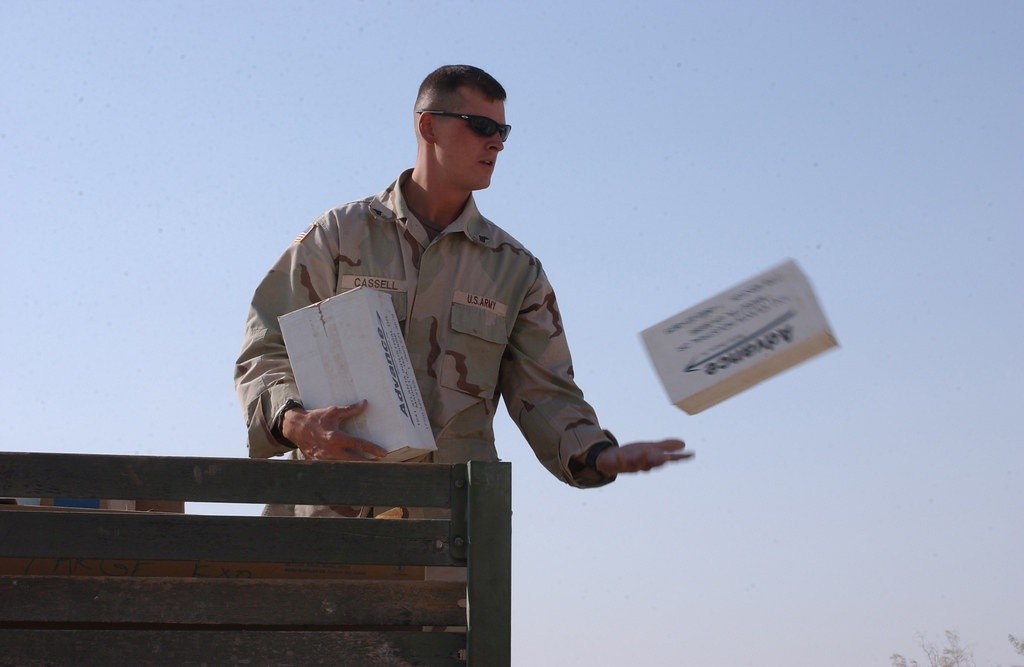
[235,65,693,521]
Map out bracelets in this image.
[586,442,611,473]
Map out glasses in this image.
[414,109,513,142]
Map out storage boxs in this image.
[639,260,839,416]
[0,504,426,631]
[277,286,438,462]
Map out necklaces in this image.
[403,182,444,234]
